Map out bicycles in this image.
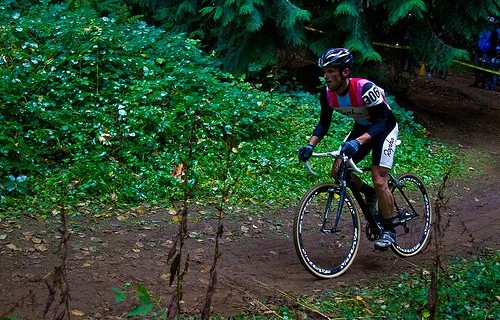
[292,141,433,279]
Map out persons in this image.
[298,48,398,251]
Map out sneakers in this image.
[375,229,396,247]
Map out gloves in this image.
[298,144,315,157]
[341,140,360,152]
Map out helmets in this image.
[318,48,352,68]
